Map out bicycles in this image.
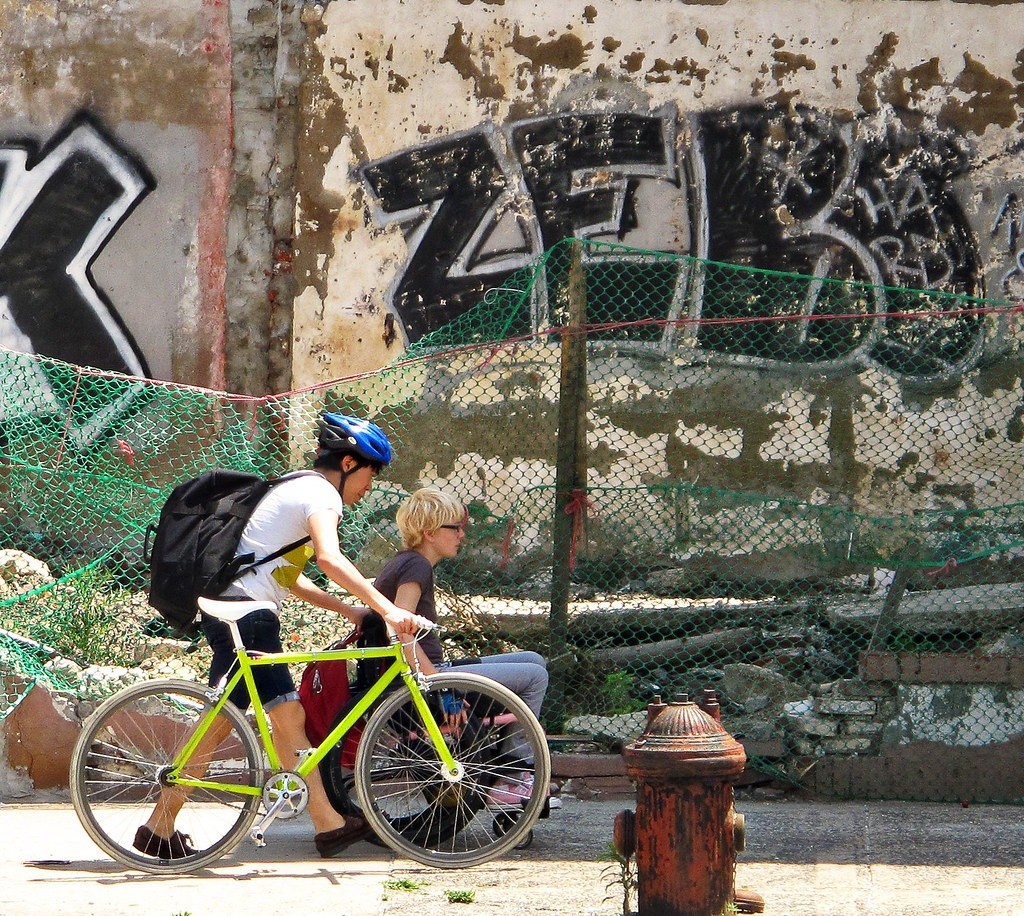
[68,592,554,876]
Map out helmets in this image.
[319,412,391,466]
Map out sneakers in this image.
[486,783,563,812]
[522,771,559,793]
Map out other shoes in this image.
[314,809,390,857]
[133,825,206,860]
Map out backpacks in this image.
[143,469,326,632]
[298,629,367,770]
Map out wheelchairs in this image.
[319,631,550,860]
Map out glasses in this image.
[441,524,461,532]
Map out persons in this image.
[133,412,420,859]
[356,487,565,813]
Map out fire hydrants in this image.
[614,688,764,916]
